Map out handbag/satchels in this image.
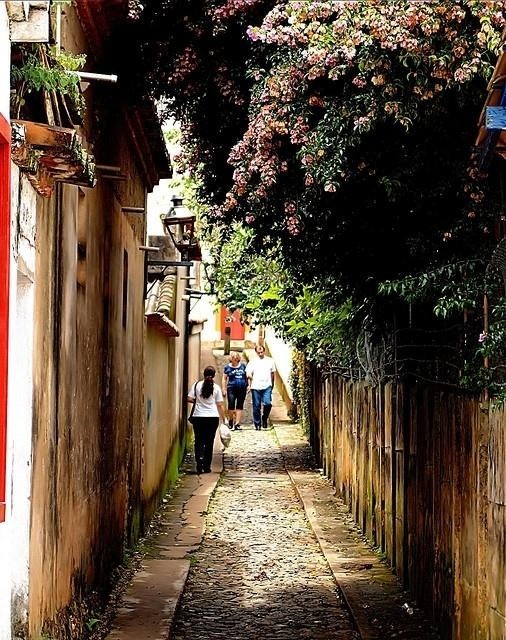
[188,399,196,423]
[221,424,231,448]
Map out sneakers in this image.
[262,416,267,428]
[256,424,261,430]
[235,424,242,430]
[229,420,233,429]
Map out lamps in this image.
[143,194,196,299]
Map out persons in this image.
[243,345,276,431]
[186,366,230,475]
[221,353,250,431]
